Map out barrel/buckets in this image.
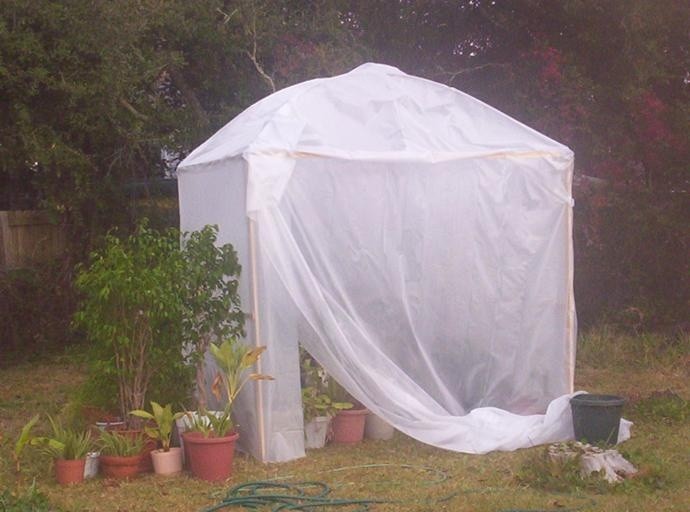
[175,410,231,467]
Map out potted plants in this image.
[299,335,368,450]
[28,411,92,487]
[73,218,276,480]
